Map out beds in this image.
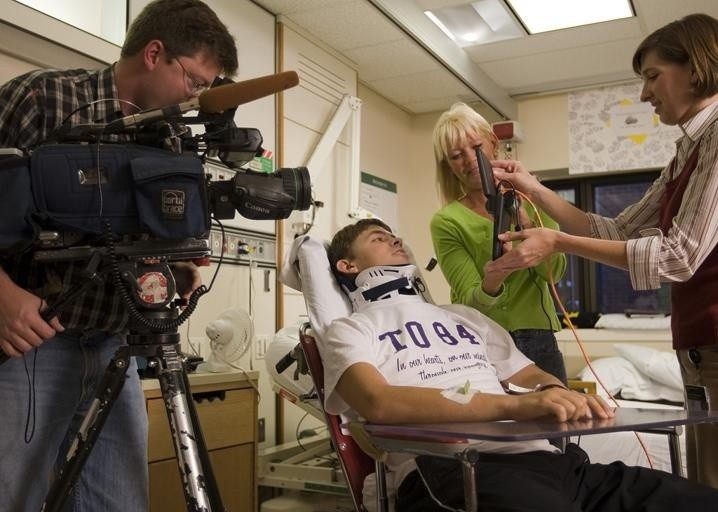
[266,321,688,479]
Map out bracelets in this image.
[540,384,568,392]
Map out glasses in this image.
[176,58,204,94]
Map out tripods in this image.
[35,343,225,511]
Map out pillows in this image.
[613,338,684,390]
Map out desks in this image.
[364,398,718,512]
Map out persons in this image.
[0,0,238,512]
[323,218,718,512]
[430,101,571,454]
[486,13,718,489]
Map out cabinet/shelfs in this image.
[140,372,262,511]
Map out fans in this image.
[200,306,256,373]
